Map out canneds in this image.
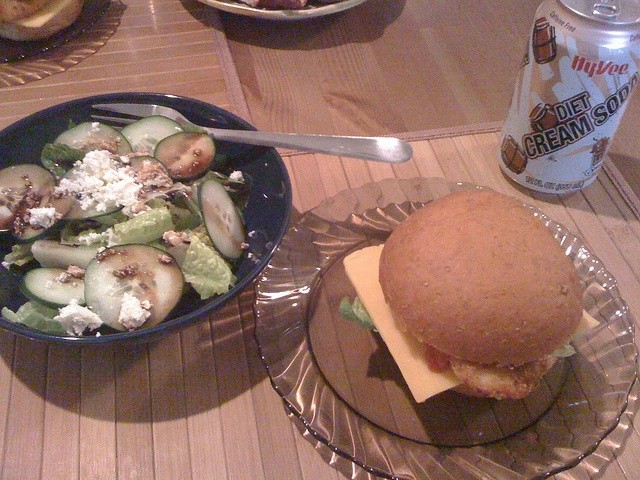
[497,1,640,194]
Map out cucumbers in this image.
[1,115,246,333]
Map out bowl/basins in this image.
[0,90,295,349]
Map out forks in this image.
[90,102,413,170]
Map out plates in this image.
[203,1,377,27]
[254,176,639,480]
[1,1,115,63]
[0,0,125,90]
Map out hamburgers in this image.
[342,189,601,409]
